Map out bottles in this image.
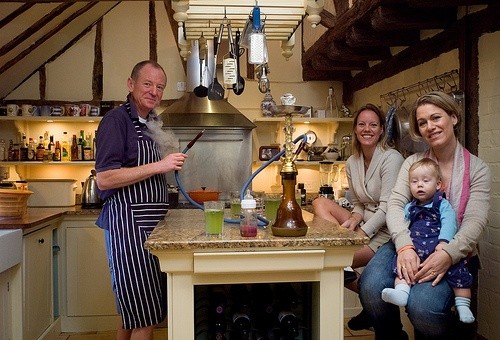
[0,128,98,161]
[326,86,339,118]
[261,94,275,117]
[281,92,297,105]
[239,188,258,236]
[295,182,335,206]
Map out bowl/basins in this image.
[302,146,339,161]
[0,189,33,218]
[269,105,310,117]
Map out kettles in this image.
[81,168,102,209]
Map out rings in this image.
[402,265,406,269]
[432,272,437,277]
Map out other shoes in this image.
[344,269,357,284]
[347,309,371,331]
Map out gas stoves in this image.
[167,203,232,209]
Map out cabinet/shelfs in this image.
[252,117,355,163]
[0,215,119,339]
[0,116,103,165]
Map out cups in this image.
[51,105,65,116]
[79,104,91,116]
[22,105,37,116]
[7,104,19,116]
[64,104,81,116]
[41,105,55,116]
[251,192,266,214]
[204,200,223,235]
[89,106,100,117]
[229,191,240,218]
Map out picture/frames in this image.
[259,146,279,161]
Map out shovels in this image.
[208,23,225,101]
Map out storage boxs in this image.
[26,179,77,206]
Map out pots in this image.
[186,186,222,205]
[166,183,179,205]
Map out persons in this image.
[357,91,491,340]
[312,103,405,336]
[95,61,188,340]
[382,157,475,324]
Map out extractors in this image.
[158,40,257,130]
